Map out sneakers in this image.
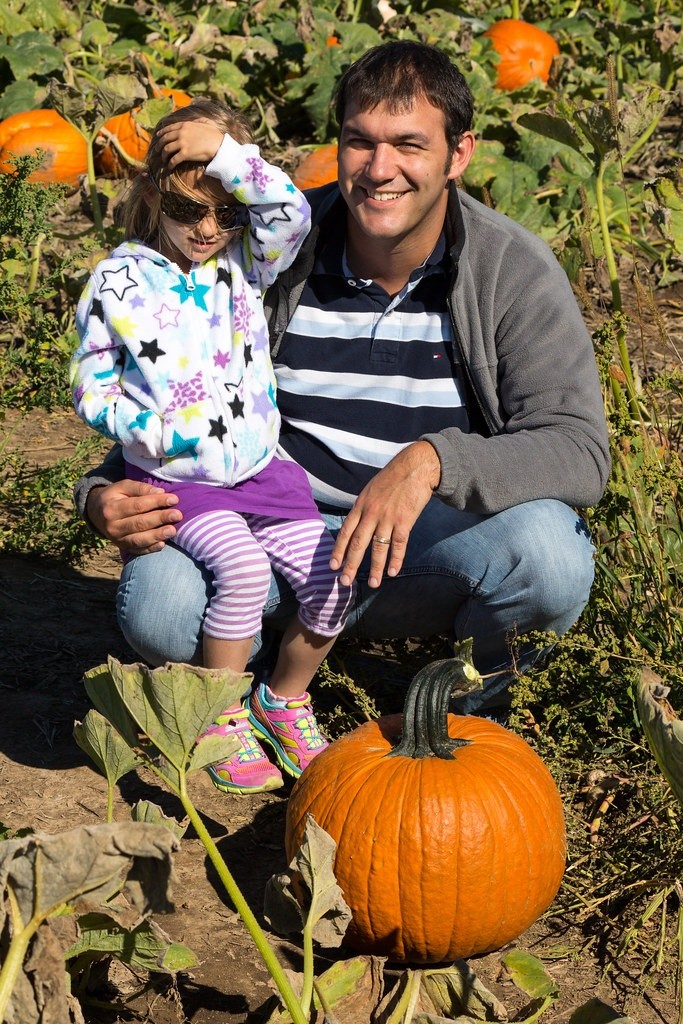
[194,708,284,795]
[241,674,331,779]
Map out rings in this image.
[373,536,391,544]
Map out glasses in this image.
[146,163,250,232]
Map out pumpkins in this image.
[290,144,340,190]
[477,18,563,93]
[286,661,566,964]
[0,85,193,197]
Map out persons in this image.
[73,39,610,716]
[69,100,358,794]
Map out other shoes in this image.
[448,704,508,727]
[245,630,283,690]
